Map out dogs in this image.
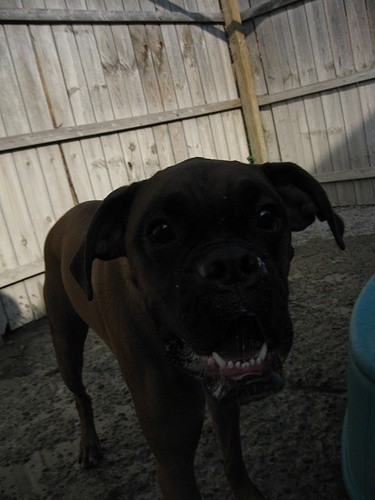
[42,156,346,500]
